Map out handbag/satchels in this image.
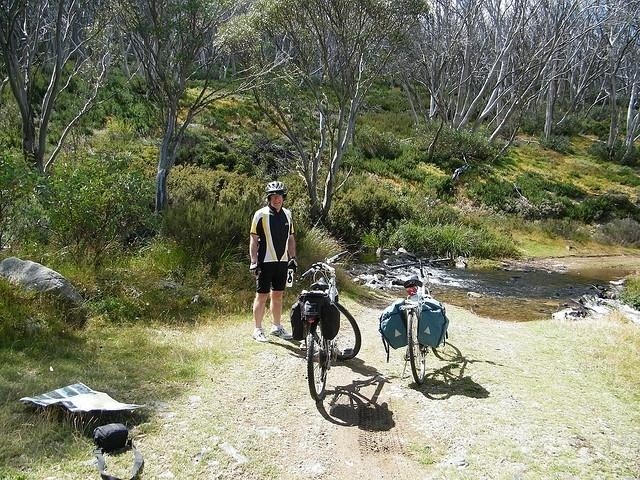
[416,295,449,349]
[378,297,409,350]
[288,291,340,341]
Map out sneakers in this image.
[252,327,271,342]
[271,324,292,339]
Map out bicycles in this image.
[380,255,454,387]
[300,250,362,402]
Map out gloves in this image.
[249,261,262,278]
[287,256,299,273]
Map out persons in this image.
[248,179,298,344]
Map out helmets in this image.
[265,181,287,198]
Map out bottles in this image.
[285,265,296,289]
